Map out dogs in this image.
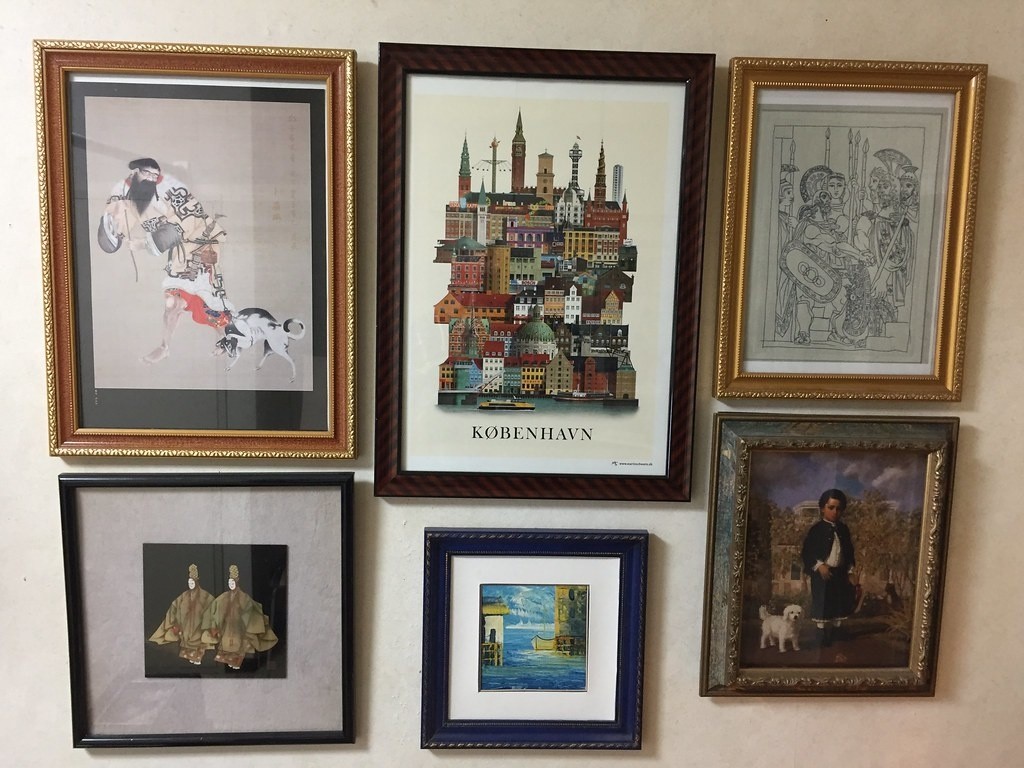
[884,583,904,610]
[759,604,804,652]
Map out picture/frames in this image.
[419,525,649,751]
[698,411,961,701]
[32,37,359,461]
[712,55,988,400]
[372,40,715,502]
[56,471,359,748]
